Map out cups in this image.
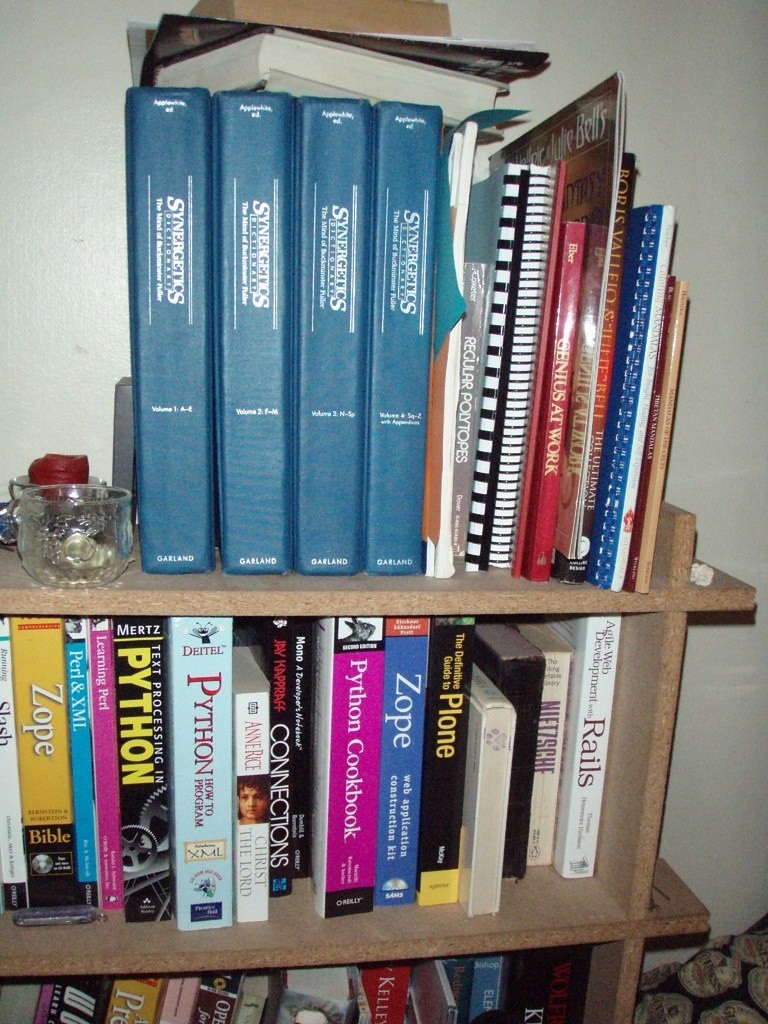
[17,483,134,587]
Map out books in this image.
[0,614,621,932]
[122,1,692,595]
[0,944,593,1024]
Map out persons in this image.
[238,778,268,825]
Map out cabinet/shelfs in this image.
[0,497,756,1024]
